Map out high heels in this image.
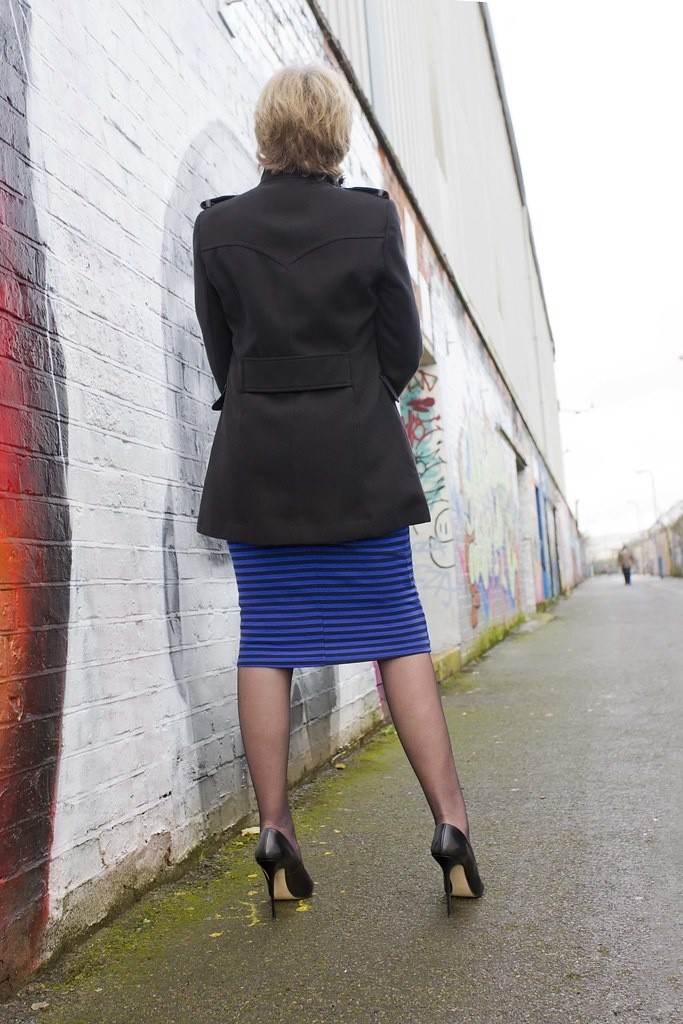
[254,828,313,922]
[431,823,484,918]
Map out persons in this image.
[191,63,485,920]
[617,543,634,586]
